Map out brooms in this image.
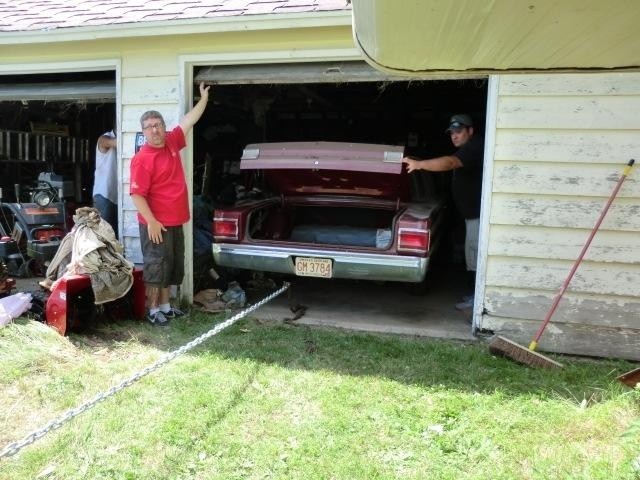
[488,158,635,374]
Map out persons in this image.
[399,111,484,312]
[91,117,118,236]
[128,81,212,324]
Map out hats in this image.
[446,113,475,132]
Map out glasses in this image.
[141,124,164,131]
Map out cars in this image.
[212,142,445,296]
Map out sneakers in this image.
[146,307,185,326]
[454,293,474,324]
